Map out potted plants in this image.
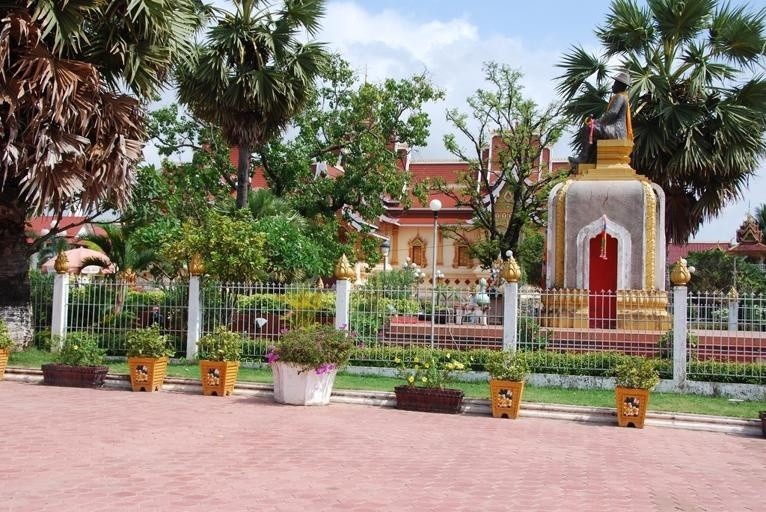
[0,314,244,398]
[610,354,661,428]
[479,349,533,419]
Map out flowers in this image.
[394,349,474,389]
[266,321,366,375]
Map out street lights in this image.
[429,199,443,351]
[380,242,389,285]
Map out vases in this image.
[393,385,464,414]
[271,360,336,406]
